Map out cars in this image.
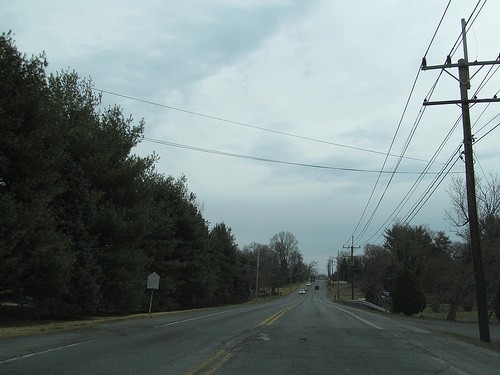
[304,275,323,291]
[299,289,305,294]
